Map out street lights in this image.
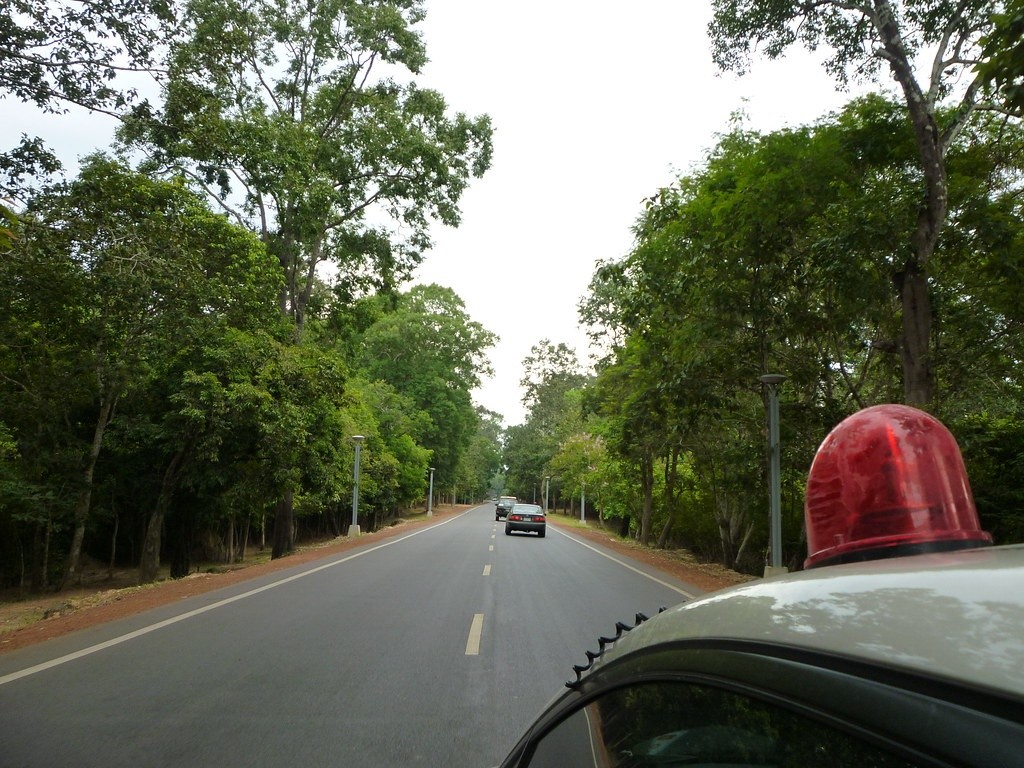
[427,468,436,511]
[545,476,550,512]
[352,436,364,525]
[759,367,788,566]
[533,483,537,501]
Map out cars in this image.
[506,504,547,537]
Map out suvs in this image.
[495,500,518,521]
